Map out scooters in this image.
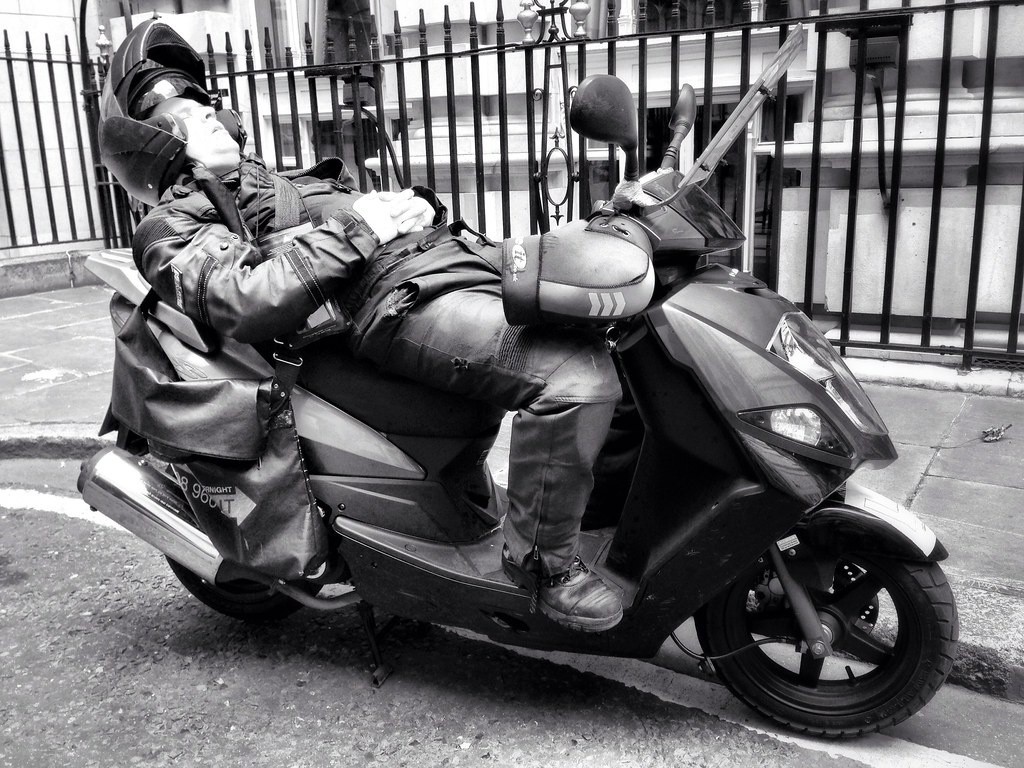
[62,20,961,743]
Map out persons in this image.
[98,18,625,631]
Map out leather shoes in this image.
[501,541,622,630]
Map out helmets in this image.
[96,20,248,207]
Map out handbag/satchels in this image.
[116,308,328,580]
